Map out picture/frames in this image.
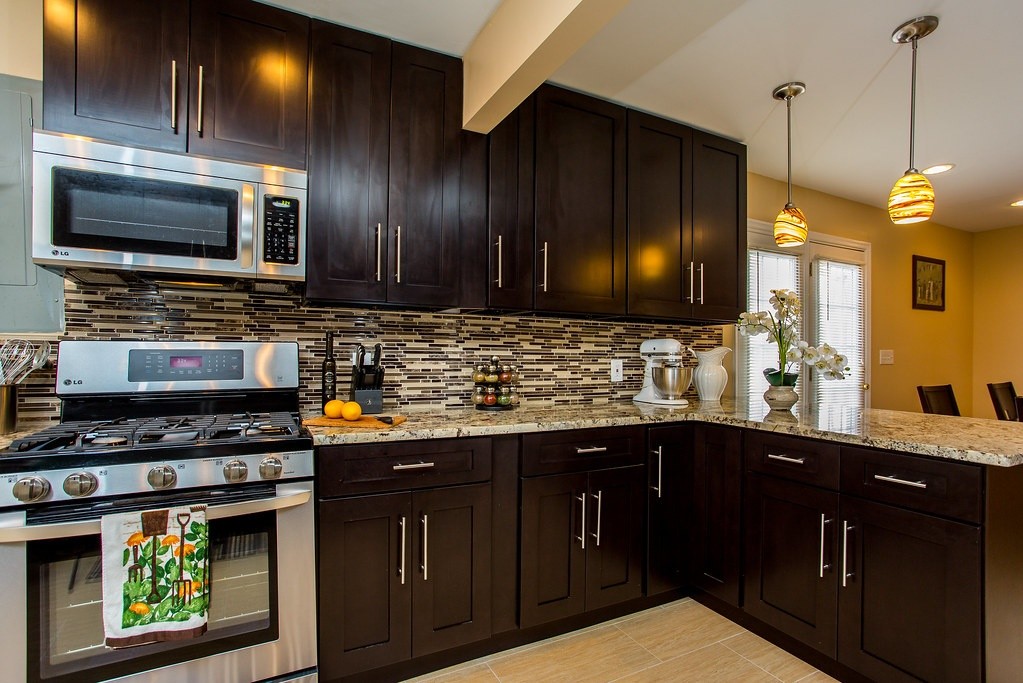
[912,253,947,310]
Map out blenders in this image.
[632,339,693,405]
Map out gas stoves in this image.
[0,341,314,509]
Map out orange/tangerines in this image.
[341,402,361,420]
[325,400,344,418]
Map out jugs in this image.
[691,346,733,401]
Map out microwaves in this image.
[33,132,308,282]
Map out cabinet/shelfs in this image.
[745,428,1022,682]
[509,424,647,627]
[629,106,751,323]
[308,16,481,305]
[487,79,631,313]
[645,424,694,599]
[689,423,744,610]
[315,437,498,683]
[45,1,306,172]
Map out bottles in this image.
[471,364,519,405]
[321,331,336,414]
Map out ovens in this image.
[0,481,318,683]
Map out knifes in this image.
[356,343,383,373]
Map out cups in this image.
[0,385,18,434]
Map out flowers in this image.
[736,288,850,382]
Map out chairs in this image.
[987,381,1017,422]
[917,385,960,417]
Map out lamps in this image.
[889,14,939,226]
[770,77,807,247]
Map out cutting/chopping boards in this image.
[302,415,407,429]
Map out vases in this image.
[763,368,799,412]
